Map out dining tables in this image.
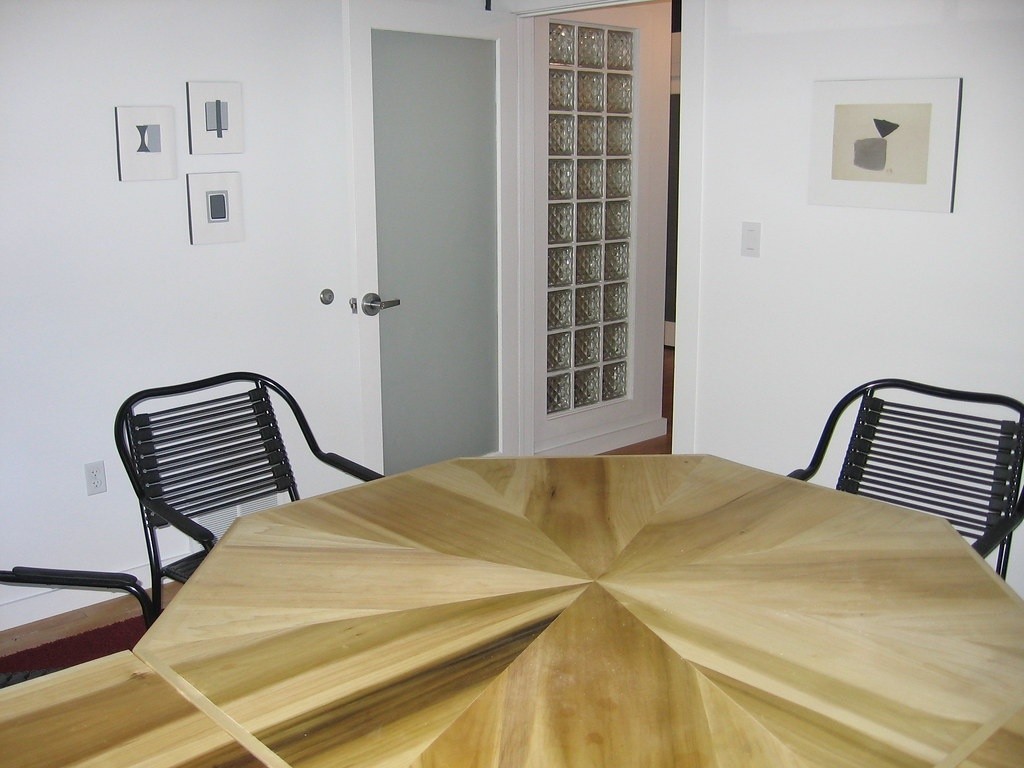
[1,455,1024,767]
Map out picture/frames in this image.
[188,172,245,245]
[116,104,179,180]
[812,78,962,213]
[187,81,242,154]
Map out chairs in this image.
[787,379,1024,580]
[115,372,386,618]
[1,567,155,630]
